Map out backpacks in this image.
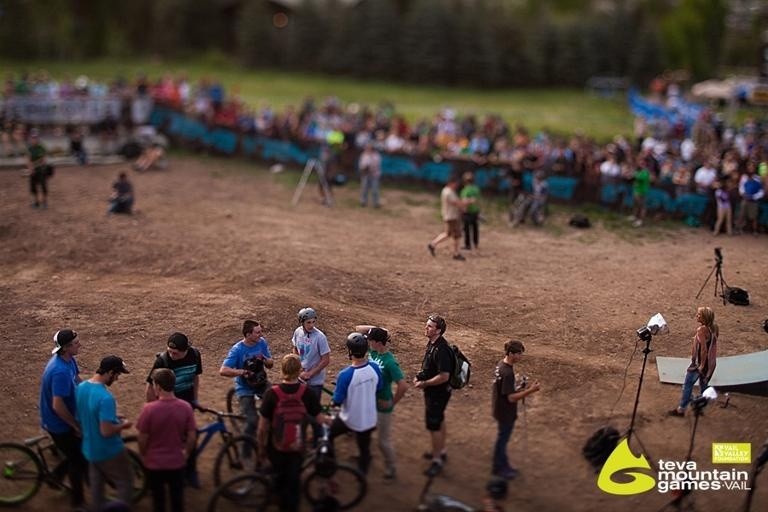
[271,383,308,454]
[428,340,472,389]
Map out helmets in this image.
[346,331,369,356]
[298,307,318,324]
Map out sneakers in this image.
[490,468,520,482]
[427,244,437,257]
[627,215,758,239]
[42,473,63,493]
[423,450,447,462]
[383,467,396,479]
[453,254,466,261]
[424,462,443,477]
[666,408,684,417]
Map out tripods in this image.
[696,260,726,306]
[619,340,659,475]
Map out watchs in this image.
[422,381,426,387]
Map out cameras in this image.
[416,371,427,380]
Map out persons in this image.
[482,480,511,511]
[414,313,455,477]
[490,341,541,478]
[667,307,718,418]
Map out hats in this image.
[99,355,131,375]
[166,332,193,351]
[364,327,389,341]
[51,328,77,355]
